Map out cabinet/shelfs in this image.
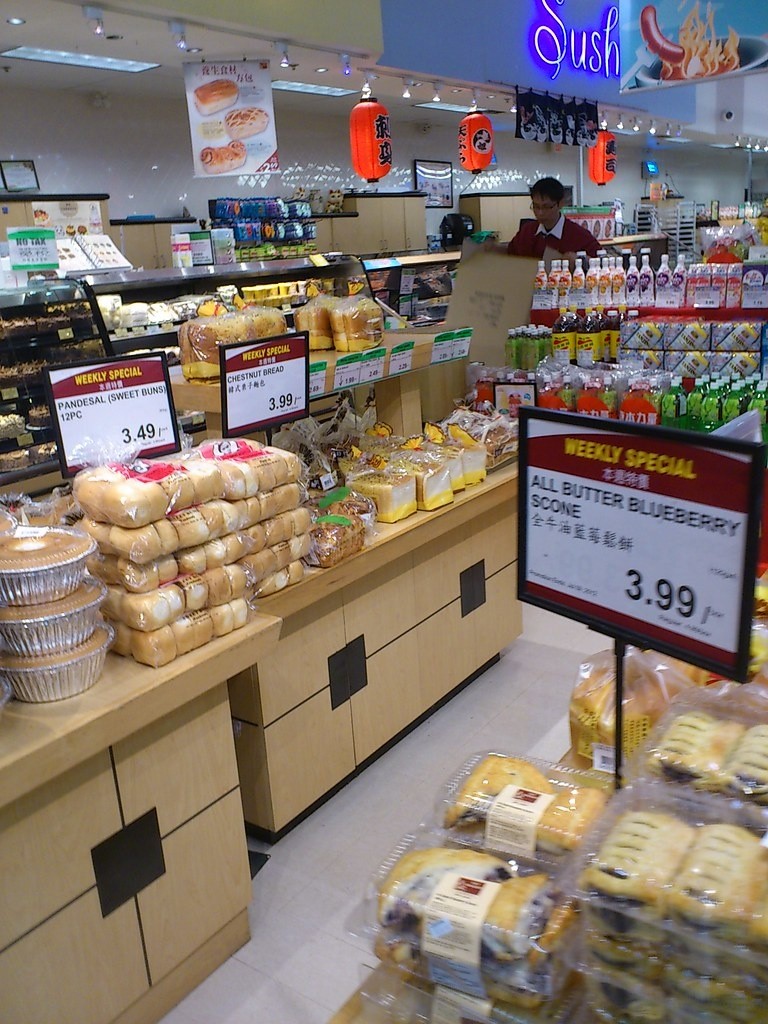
[0,248,461,502]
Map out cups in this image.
[241,282,307,308]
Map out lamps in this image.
[86,18,681,137]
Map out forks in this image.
[620,35,674,90]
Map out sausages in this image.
[640,5,685,63]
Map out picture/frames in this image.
[413,158,455,208]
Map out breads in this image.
[294,303,382,350]
[177,308,287,381]
[305,417,518,567]
[194,79,239,117]
[226,108,270,140]
[0,514,108,704]
[201,141,245,173]
[373,639,768,1024]
[74,438,310,667]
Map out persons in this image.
[507,177,603,273]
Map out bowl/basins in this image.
[635,37,768,91]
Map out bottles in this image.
[535,367,768,445]
[531,249,688,309]
[504,306,639,371]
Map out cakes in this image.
[0,314,71,470]
